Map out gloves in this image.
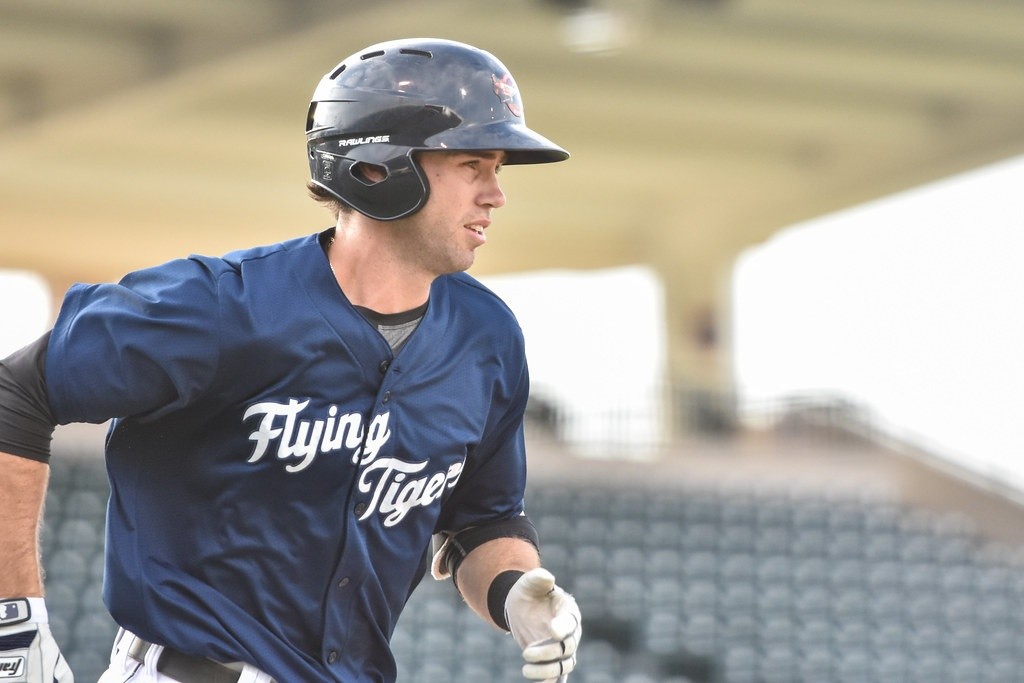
[0,597,74,683]
[486,569,582,683]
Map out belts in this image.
[117,630,240,683]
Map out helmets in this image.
[305,38,570,222]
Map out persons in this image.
[0,36,582,683]
